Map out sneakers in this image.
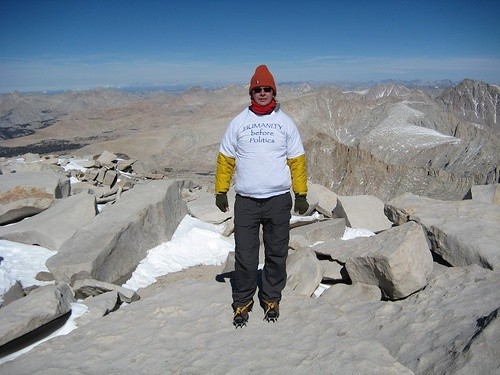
[262,300,279,318]
[234,301,253,323]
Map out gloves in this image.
[294,196,309,215]
[216,194,228,212]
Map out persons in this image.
[215,65,309,327]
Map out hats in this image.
[249,65,276,95]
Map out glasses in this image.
[254,87,271,93]
